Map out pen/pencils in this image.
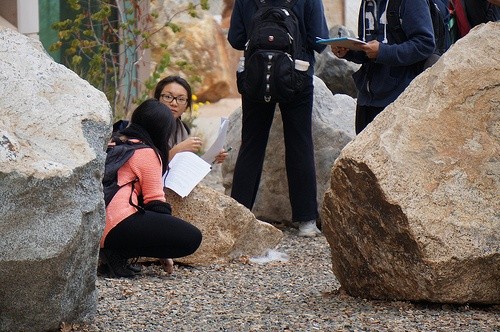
[337,27,343,57]
[212,147,232,163]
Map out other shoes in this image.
[298,220,321,237]
[102,258,136,279]
[127,265,142,272]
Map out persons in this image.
[331,0,436,135]
[426,0,500,70]
[101,98,202,277]
[228,0,329,237]
[105,74,229,162]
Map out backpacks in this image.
[424,0,457,70]
[235,0,310,102]
[103,135,151,215]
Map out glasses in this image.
[160,94,189,105]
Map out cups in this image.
[295,59,310,71]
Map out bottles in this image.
[237,57,245,72]
